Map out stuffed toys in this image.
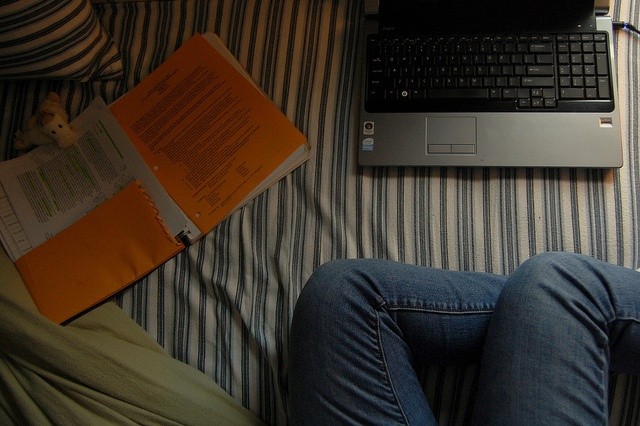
[15,91,87,149]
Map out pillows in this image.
[0,1,125,80]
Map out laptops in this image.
[357,0,623,167]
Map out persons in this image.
[284,248,639,426]
